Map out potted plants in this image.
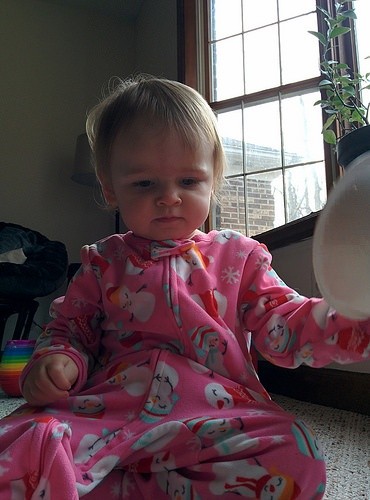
[306,0,370,172]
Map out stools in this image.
[0,299,39,359]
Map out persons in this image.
[0,77,370,500]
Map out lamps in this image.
[70,130,121,237]
